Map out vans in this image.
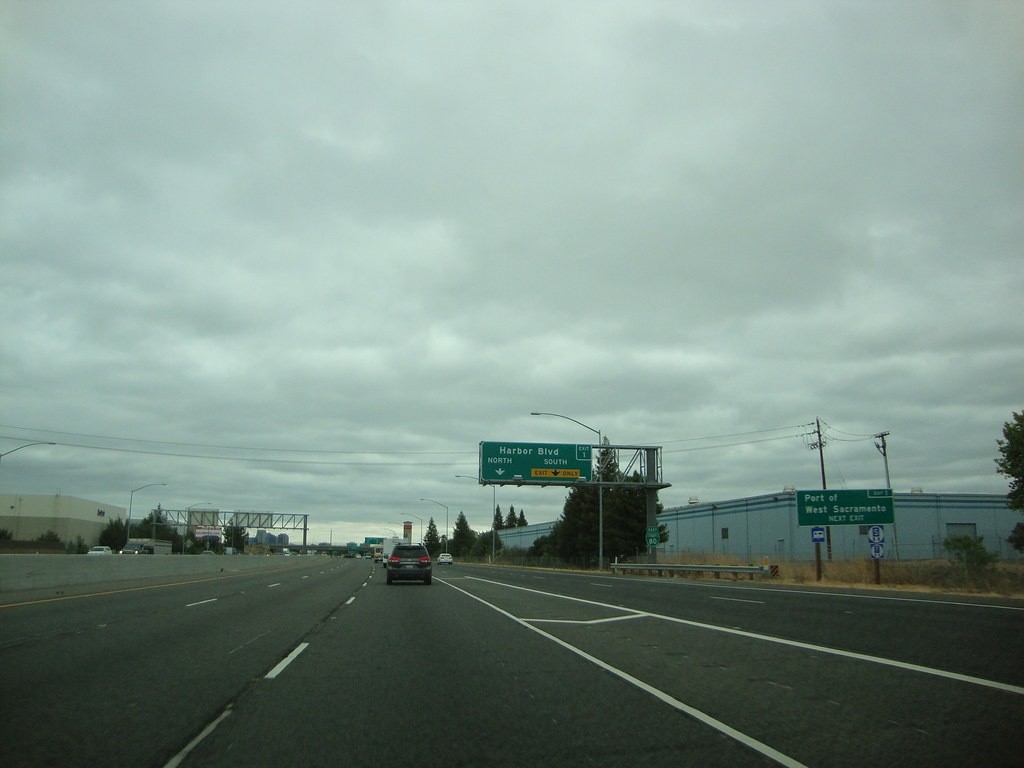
[374,546,384,562]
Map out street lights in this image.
[420,499,448,553]
[382,513,423,543]
[455,475,496,560]
[127,483,167,543]
[183,502,212,555]
[531,413,603,570]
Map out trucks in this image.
[120,539,173,554]
[383,538,408,568]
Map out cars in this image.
[387,542,433,584]
[366,555,371,559]
[356,554,361,559]
[88,546,112,555]
[437,554,453,565]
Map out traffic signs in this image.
[479,441,592,484]
